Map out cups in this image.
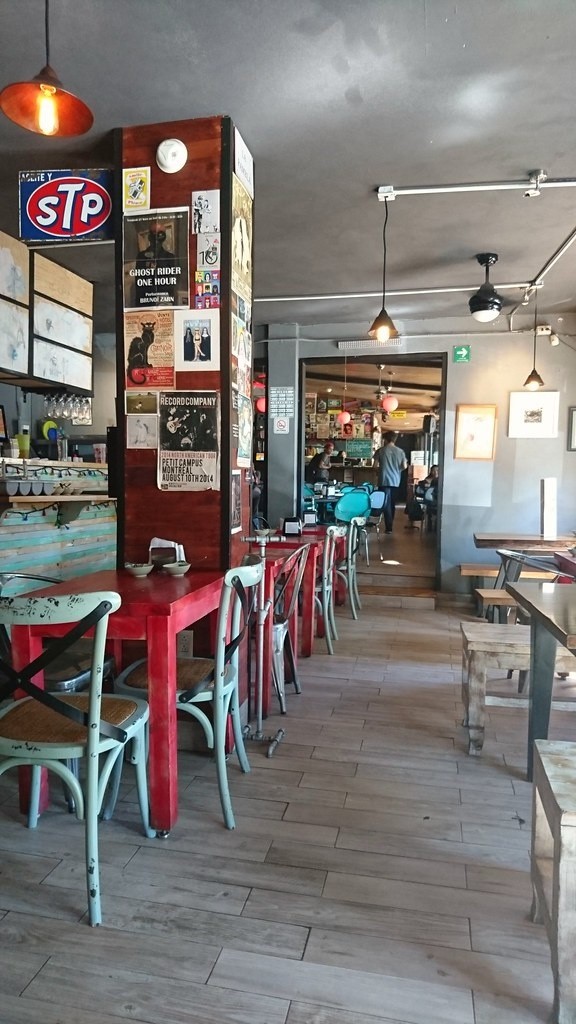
[15,434,31,459]
[92,444,107,463]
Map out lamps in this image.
[468,252,504,323]
[339,391,350,425]
[368,195,397,340]
[522,288,544,391]
[257,364,265,413]
[383,370,397,411]
[0,0,94,136]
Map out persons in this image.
[314,442,334,484]
[161,405,217,452]
[424,465,440,486]
[372,432,408,534]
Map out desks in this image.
[276,526,349,637]
[252,536,341,655]
[316,498,339,524]
[505,581,576,781]
[251,548,301,718]
[474,532,576,623]
[11,568,235,838]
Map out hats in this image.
[324,443,334,448]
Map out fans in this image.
[346,361,425,398]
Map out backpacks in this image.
[305,453,322,483]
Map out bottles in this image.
[72,443,80,461]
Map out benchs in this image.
[529,738,576,1024]
[475,588,521,679]
[456,561,558,616]
[461,620,576,757]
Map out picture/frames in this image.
[508,390,560,439]
[568,407,576,451]
[453,404,499,461]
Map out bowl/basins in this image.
[53,485,85,495]
[255,528,277,536]
[162,563,192,576]
[151,554,176,573]
[125,563,155,577]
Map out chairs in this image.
[275,524,348,655]
[0,573,121,813]
[317,516,365,620]
[306,480,437,567]
[250,542,311,716]
[105,554,264,830]
[0,591,156,929]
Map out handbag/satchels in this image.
[407,496,424,521]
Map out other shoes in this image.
[385,530,392,534]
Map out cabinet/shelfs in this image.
[1,229,95,397]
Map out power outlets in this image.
[537,325,551,335]
